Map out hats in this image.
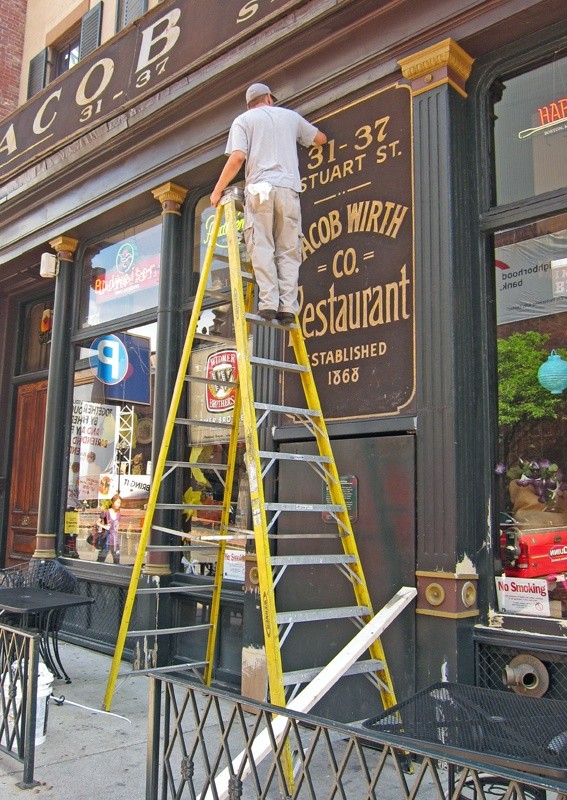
[246,83,277,105]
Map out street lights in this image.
[116,442,127,495]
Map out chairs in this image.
[0,556,76,680]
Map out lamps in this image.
[40,251,59,278]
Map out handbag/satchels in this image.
[86,510,108,550]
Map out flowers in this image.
[493,456,567,512]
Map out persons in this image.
[96,494,122,565]
[209,83,327,322]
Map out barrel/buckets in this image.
[1,672,54,748]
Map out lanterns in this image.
[537,348,567,394]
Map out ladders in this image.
[102,186,414,800]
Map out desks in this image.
[0,587,95,683]
[363,682,567,800]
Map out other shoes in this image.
[256,310,276,320]
[276,312,295,323]
